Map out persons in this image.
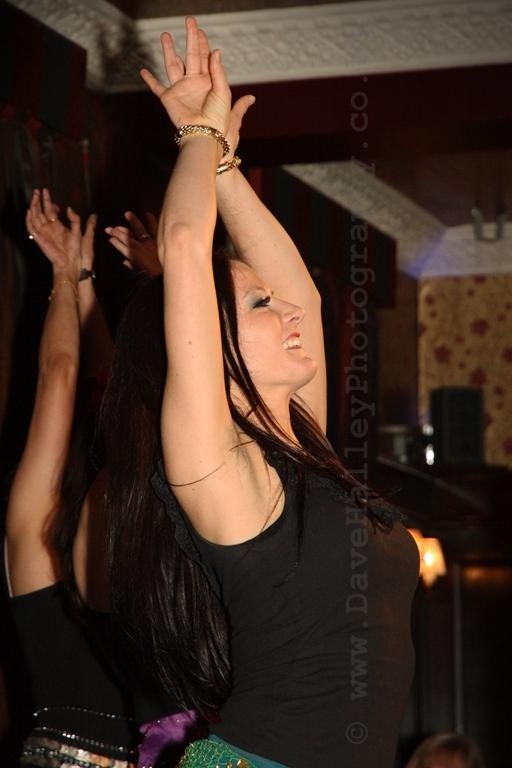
[406,734,484,768]
[5,189,155,767]
[94,17,420,768]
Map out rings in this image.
[29,231,37,239]
[48,218,57,222]
[138,232,151,240]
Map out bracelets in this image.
[48,281,78,300]
[77,268,96,281]
[216,156,242,175]
[175,125,230,156]
